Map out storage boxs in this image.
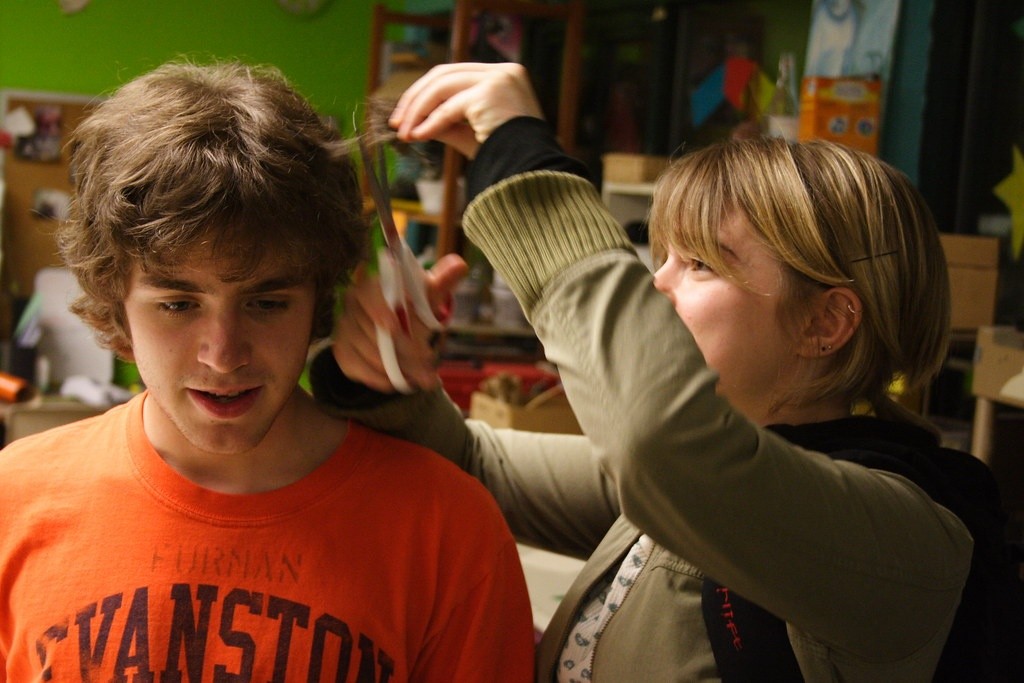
[937,233,1002,326]
[798,76,882,156]
[472,381,580,433]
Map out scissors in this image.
[351,109,454,395]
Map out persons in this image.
[0,48,538,683]
[306,60,1024,683]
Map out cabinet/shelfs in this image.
[356,1,595,377]
